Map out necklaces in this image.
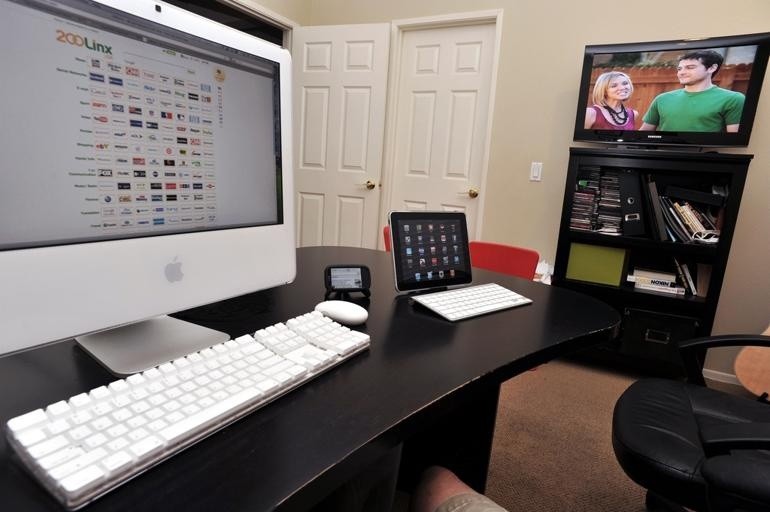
[603,101,628,124]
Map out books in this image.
[570,166,729,297]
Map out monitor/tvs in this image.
[573,32,770,153]
[0,0,298,379]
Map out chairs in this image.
[470,240,540,282]
[612,334,770,512]
[384,226,391,252]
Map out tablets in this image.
[387,209,474,293]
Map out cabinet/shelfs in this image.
[539,147,755,383]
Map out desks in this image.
[0,247,621,512]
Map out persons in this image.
[638,50,745,134]
[413,465,509,511]
[584,72,639,131]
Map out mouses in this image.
[313,297,371,325]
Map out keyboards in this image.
[410,279,534,325]
[4,306,372,512]
